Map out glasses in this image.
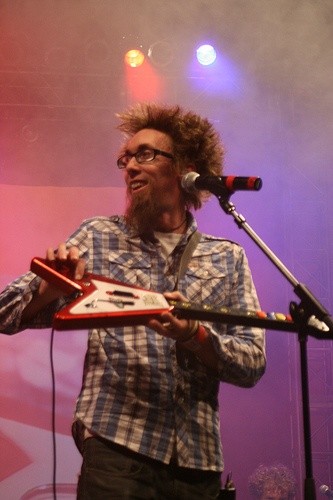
[117,147,175,169]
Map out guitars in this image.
[29,256,333,342]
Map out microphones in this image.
[180,172,263,193]
[319,484,333,497]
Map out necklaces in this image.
[156,220,187,232]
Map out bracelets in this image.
[187,319,199,337]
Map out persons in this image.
[0,100,269,500]
